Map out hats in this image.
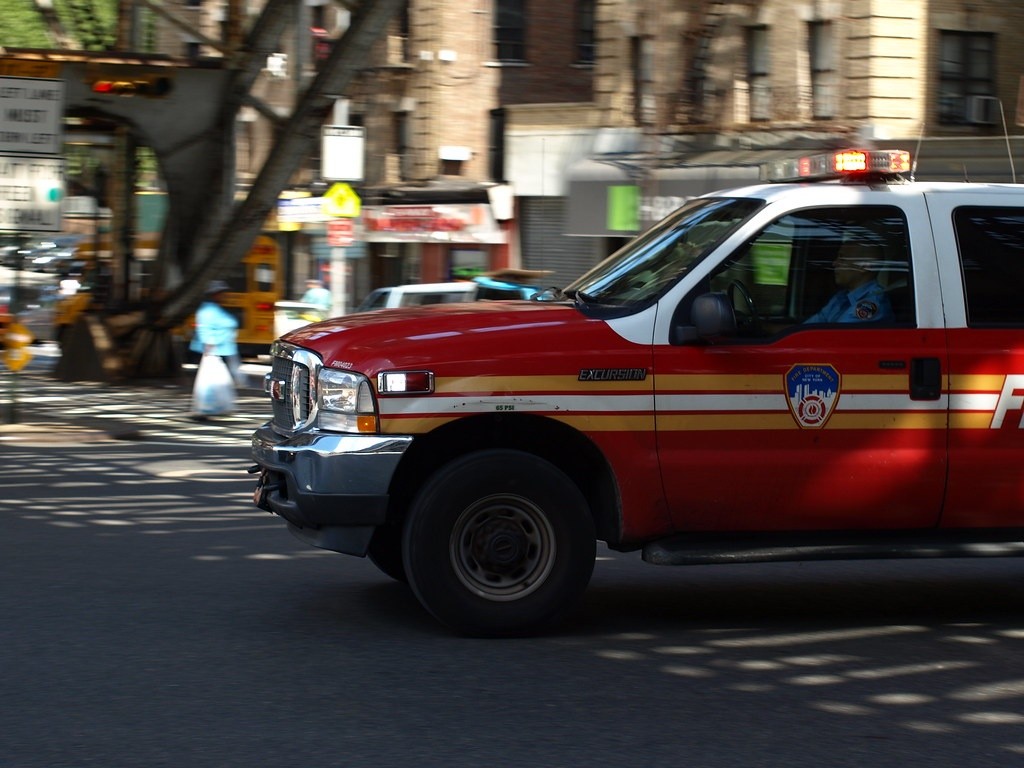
[202,280,234,294]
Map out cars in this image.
[1,234,88,346]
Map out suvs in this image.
[247,147,1024,638]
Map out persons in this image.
[300,279,334,310]
[758,237,894,337]
[189,281,240,421]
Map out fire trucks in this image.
[110,175,525,370]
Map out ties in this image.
[828,302,851,321]
